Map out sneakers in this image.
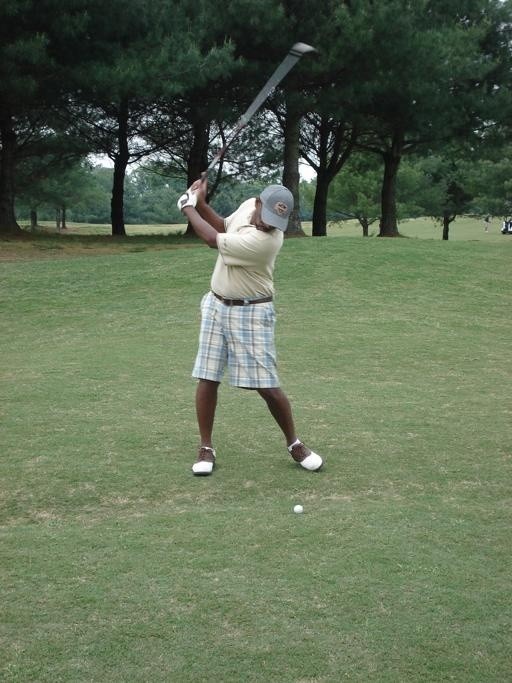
[287,438,323,471]
[192,445,216,475]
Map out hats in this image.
[259,184,295,233]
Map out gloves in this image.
[176,185,198,212]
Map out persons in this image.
[176,169,325,476]
[483,214,491,232]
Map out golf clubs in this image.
[192,42,317,192]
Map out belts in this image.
[210,288,273,306]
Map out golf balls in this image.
[294,505,303,513]
